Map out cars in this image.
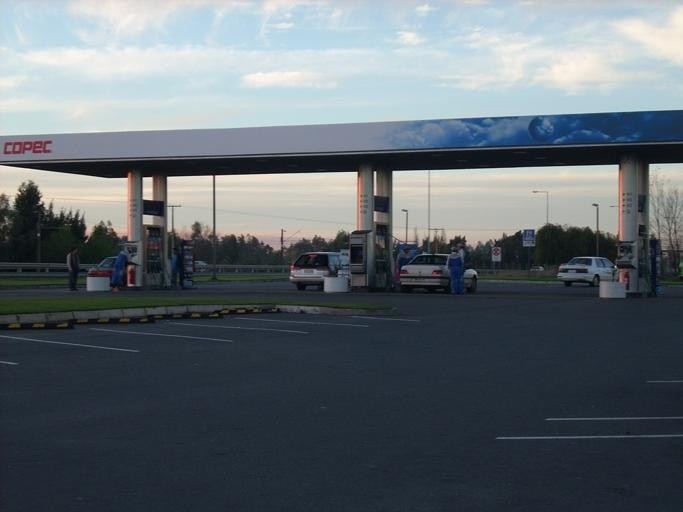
[400,253,479,293]
[194,261,209,273]
[557,256,615,288]
[529,265,544,272]
[87,255,118,277]
[289,252,340,290]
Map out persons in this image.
[455,242,465,265]
[394,245,412,274]
[170,248,183,290]
[446,246,465,294]
[110,247,133,292]
[65,246,82,291]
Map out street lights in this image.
[401,208,409,243]
[591,203,600,256]
[531,190,548,223]
